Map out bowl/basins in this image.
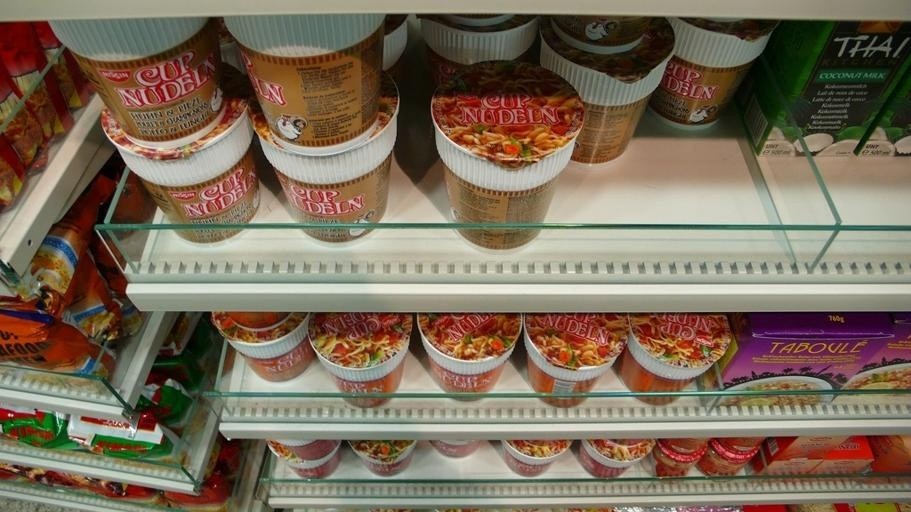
[714,374,834,403]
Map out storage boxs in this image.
[693,309,911,417]
[734,19,911,159]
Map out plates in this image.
[834,360,911,396]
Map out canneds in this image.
[652,435,767,475]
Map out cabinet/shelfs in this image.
[89,102,909,512]
[0,43,270,512]
